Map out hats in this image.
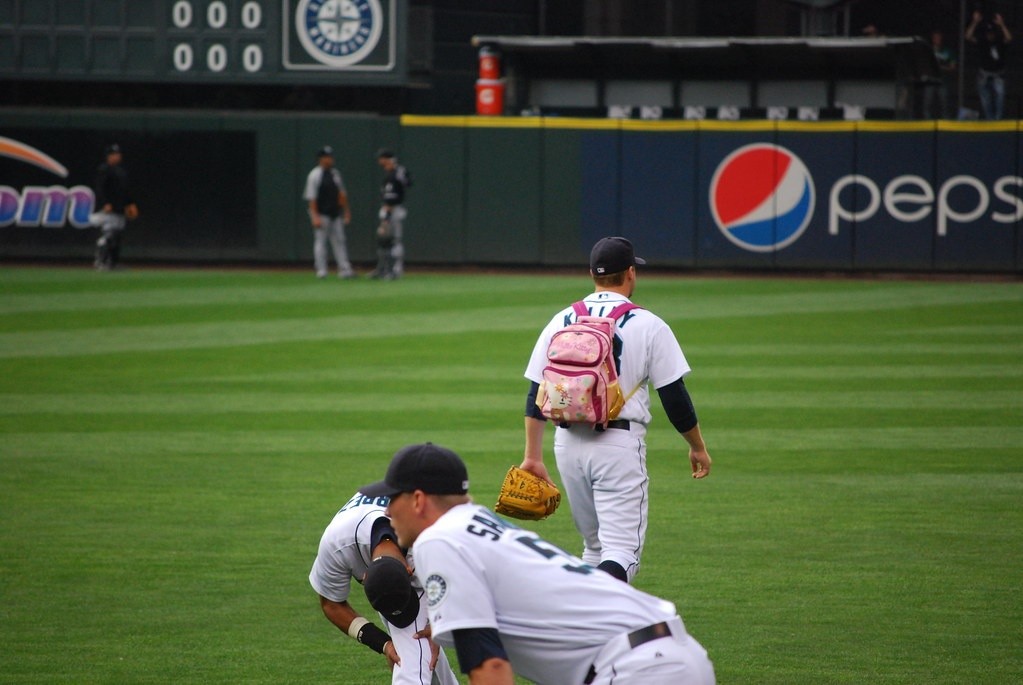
[106,143,121,155]
[358,442,469,498]
[317,145,336,157]
[590,236,646,276]
[376,147,395,158]
[364,556,420,628]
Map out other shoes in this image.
[370,271,400,280]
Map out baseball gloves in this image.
[493,464,562,521]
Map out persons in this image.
[964,11,1012,119]
[93,143,138,271]
[368,149,409,280]
[359,442,715,685]
[303,147,354,280]
[519,237,710,583]
[309,489,460,685]
[920,32,955,118]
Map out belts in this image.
[560,420,630,430]
[583,622,671,685]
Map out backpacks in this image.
[535,300,649,432]
